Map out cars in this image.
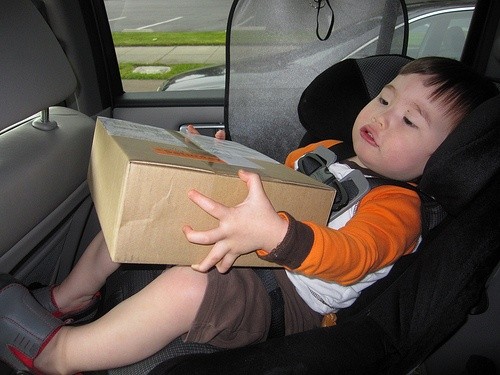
[157,2,479,102]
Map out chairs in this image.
[0,0,96,290]
[111,50,500,375]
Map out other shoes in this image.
[1,273,73,375]
[29,282,103,326]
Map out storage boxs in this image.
[87,116,336,267]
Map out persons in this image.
[0,57,482,375]
